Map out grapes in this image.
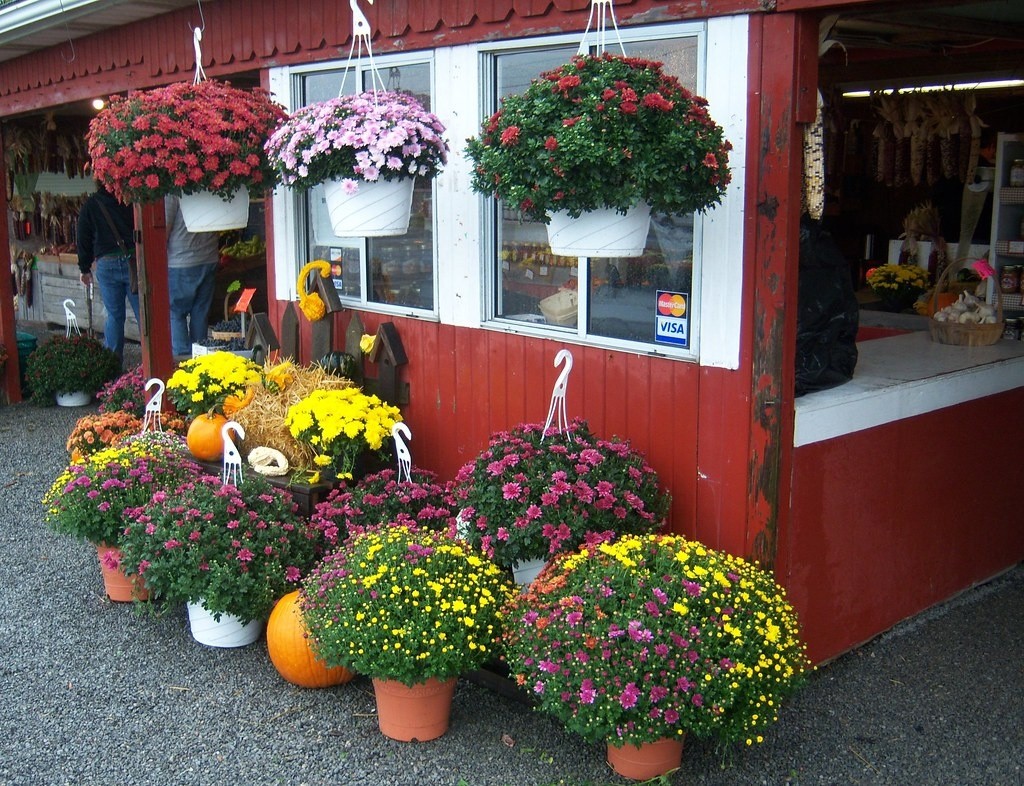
[197,315,253,353]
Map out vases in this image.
[96,539,160,601]
[55,384,91,406]
[605,728,687,780]
[175,182,251,233]
[880,288,909,312]
[542,193,654,257]
[185,595,263,649]
[324,172,417,236]
[511,554,549,595]
[370,673,457,743]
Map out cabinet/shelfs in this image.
[985,132,1024,312]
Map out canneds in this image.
[1009,159,1024,187]
[341,211,434,308]
[1000,264,1024,294]
[501,240,569,267]
[1001,315,1024,341]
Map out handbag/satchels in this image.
[128,262,138,294]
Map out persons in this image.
[164,192,219,356]
[76,177,141,365]
[793,213,860,397]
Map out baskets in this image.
[930,258,1005,346]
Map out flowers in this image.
[865,262,932,296]
[24,330,124,401]
[40,366,817,768]
[262,88,449,197]
[465,50,731,219]
[83,78,293,206]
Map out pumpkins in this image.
[187,404,234,461]
[267,589,357,688]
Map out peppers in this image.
[220,234,266,260]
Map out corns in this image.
[898,250,946,286]
[4,128,90,309]
[801,107,980,220]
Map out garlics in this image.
[933,289,998,323]
